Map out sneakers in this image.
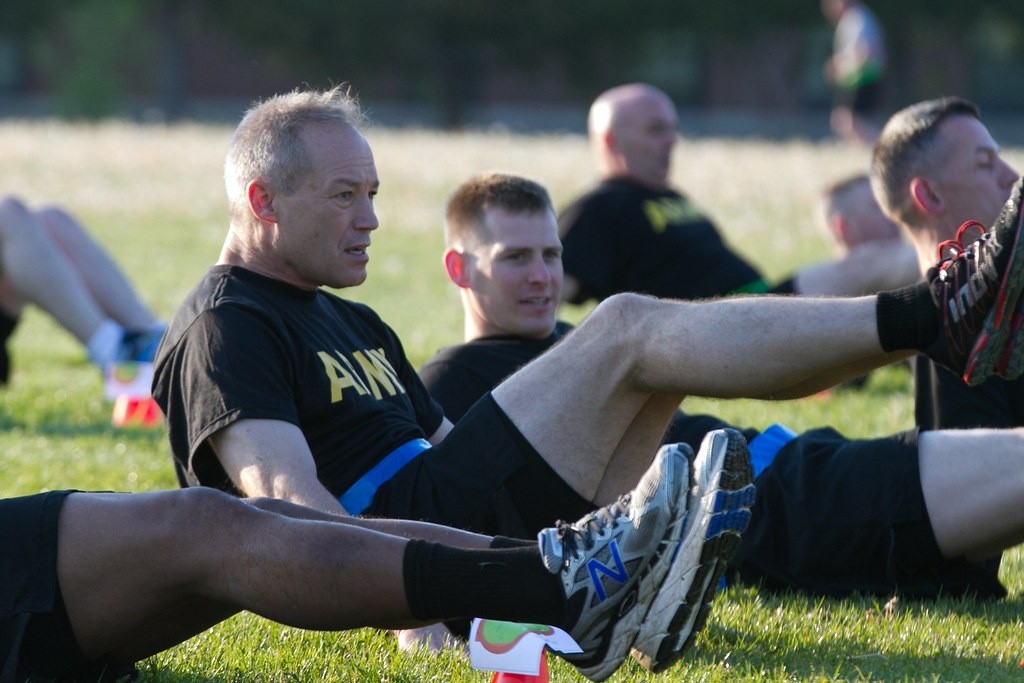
[544,443,694,682]
[931,175,1024,386]
[633,429,757,672]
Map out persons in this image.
[555,82,1024,430]
[148,91,1024,653]
[0,428,759,683]
[814,1,892,149]
[414,177,1024,605]
[1,197,170,428]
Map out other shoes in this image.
[102,326,169,401]
